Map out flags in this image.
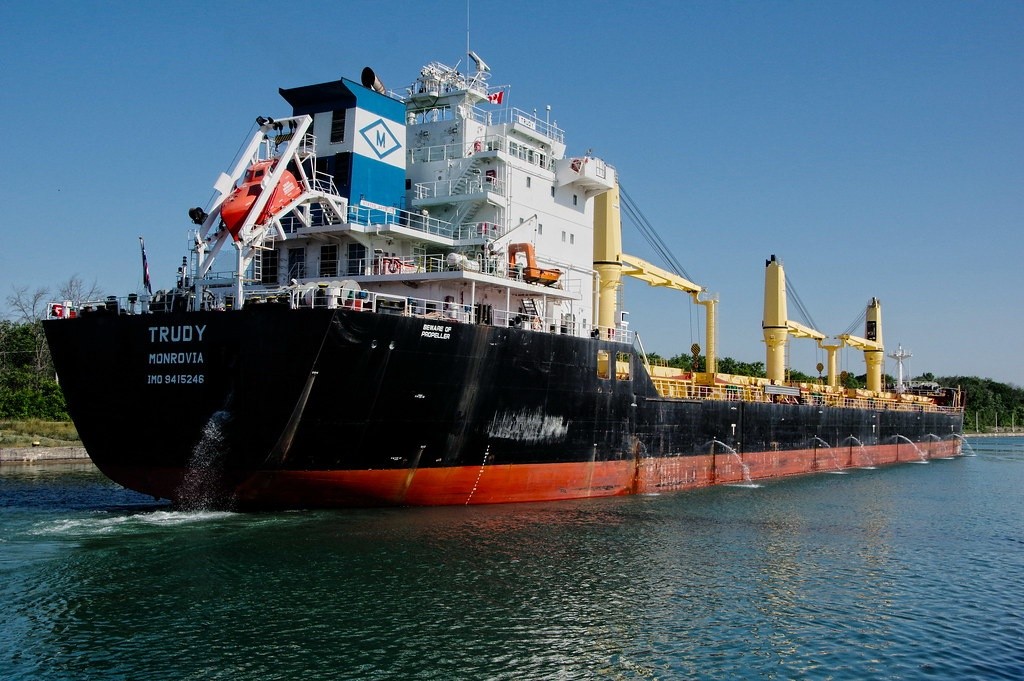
[487,91,504,104]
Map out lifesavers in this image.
[388,259,399,273]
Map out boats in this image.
[40,0,968,514]
